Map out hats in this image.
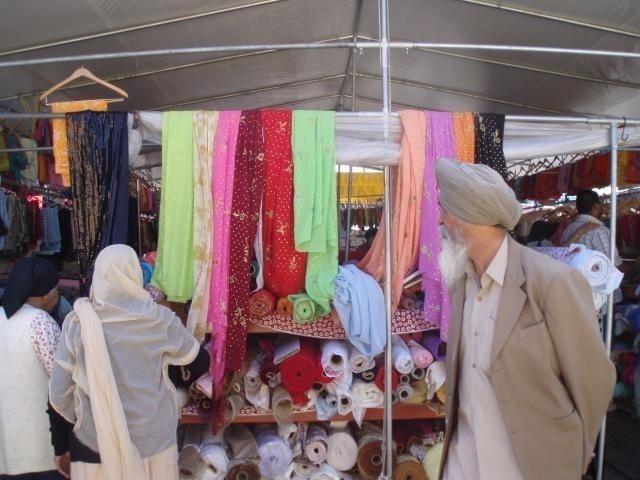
[434,156,524,231]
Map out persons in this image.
[49,244,210,480]
[435,157,617,480]
[560,189,623,304]
[0,256,61,480]
[526,220,556,247]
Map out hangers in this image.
[502,148,602,180]
[37,60,128,110]
